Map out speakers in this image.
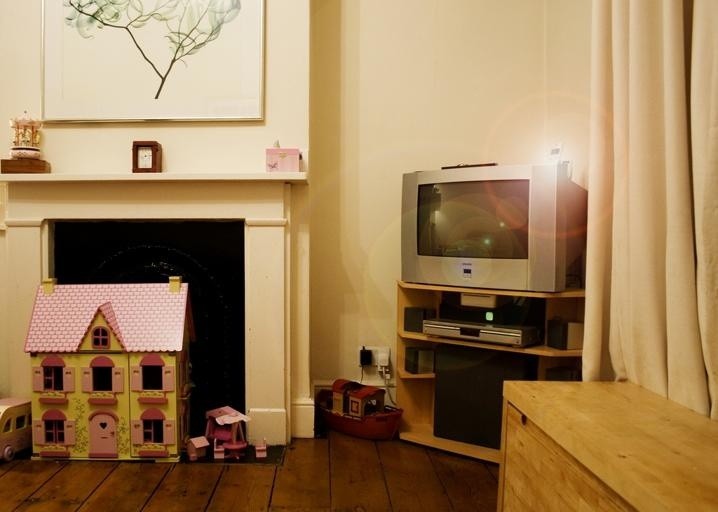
[403,307,436,333]
[405,346,436,373]
[547,321,584,350]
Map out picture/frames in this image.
[132,139,162,173]
[39,2,269,126]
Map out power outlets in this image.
[374,346,392,367]
[359,344,374,367]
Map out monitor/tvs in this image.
[400,160,588,293]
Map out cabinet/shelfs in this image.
[497,380,718,512]
[394,276,586,466]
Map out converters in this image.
[377,347,391,374]
[360,349,372,367]
